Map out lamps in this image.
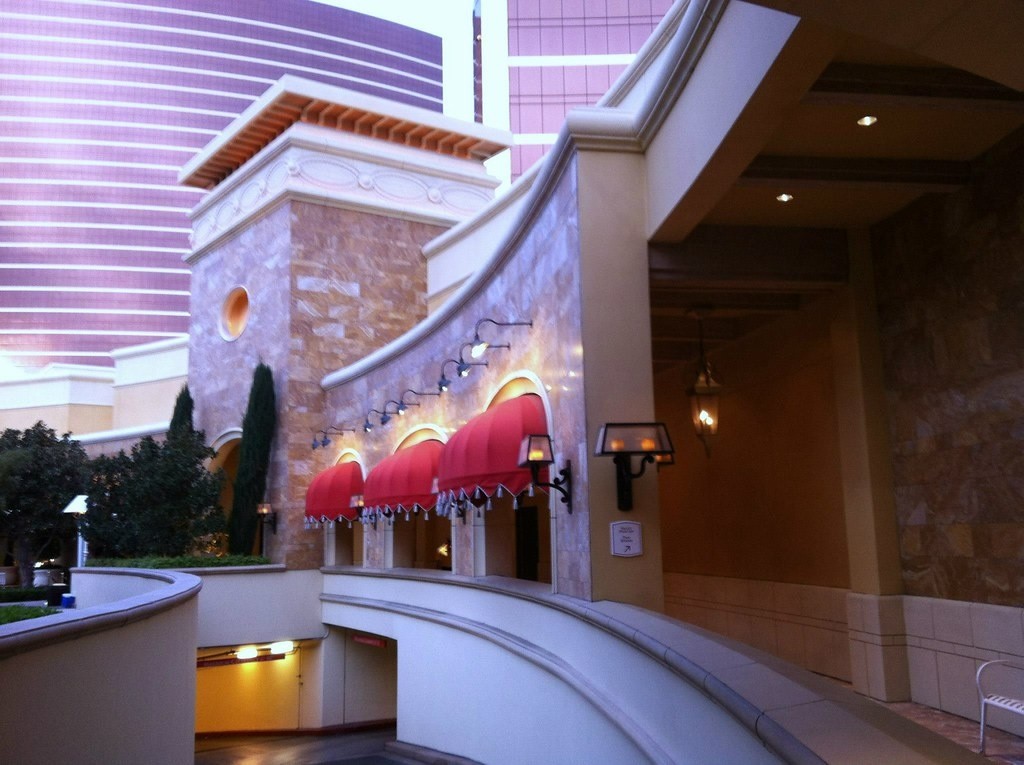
[685,304,720,458]
[312,429,344,449]
[594,422,676,511]
[321,423,355,446]
[517,433,573,514]
[437,359,488,392]
[457,344,511,376]
[398,388,443,415]
[363,408,400,433]
[380,399,419,426]
[471,318,532,357]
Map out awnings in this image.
[437,394,550,515]
[364,441,443,523]
[304,462,364,528]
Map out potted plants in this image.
[33,560,65,588]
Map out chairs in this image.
[976,659,1023,755]
